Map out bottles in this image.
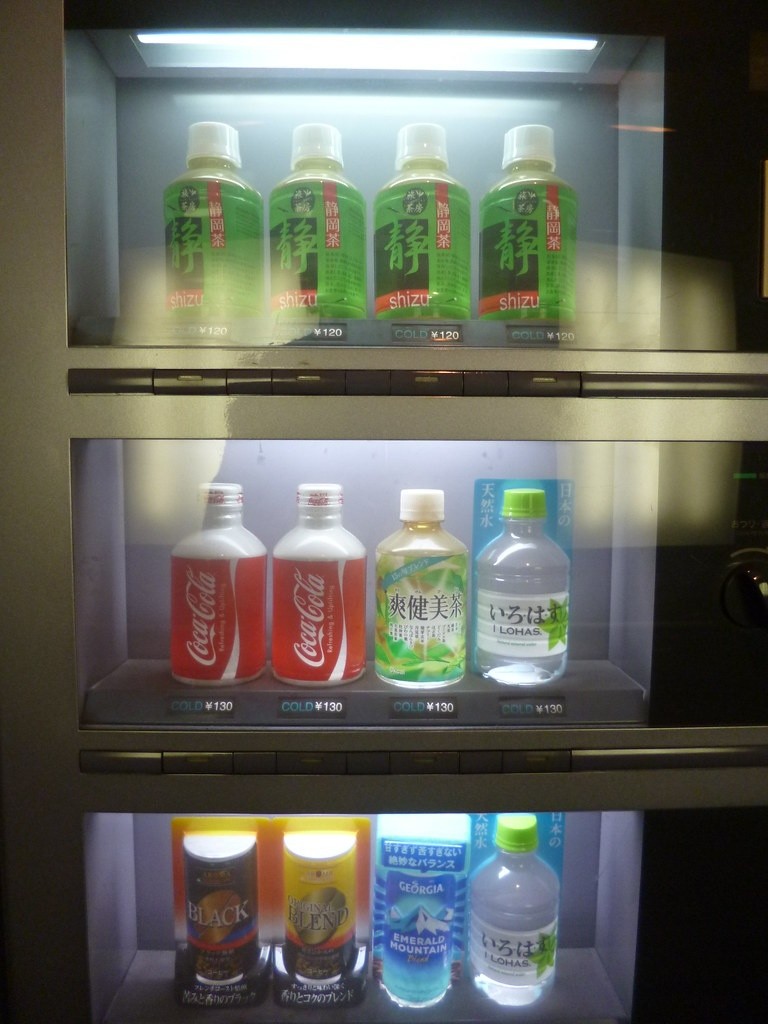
[268,122,368,323]
[162,120,266,325]
[372,123,472,317]
[270,482,369,686]
[465,812,560,1008]
[474,490,570,685]
[168,481,267,685]
[477,125,577,323]
[375,488,469,688]
